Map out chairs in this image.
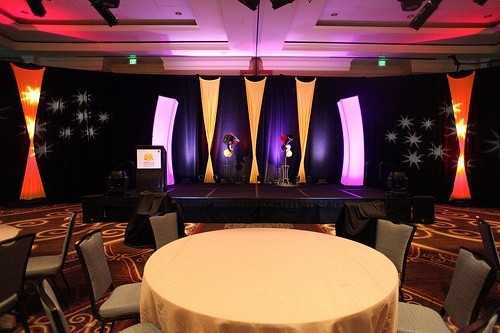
[24,211,77,310]
[36,275,161,333]
[397,245,500,333]
[477,217,500,284]
[0,233,36,333]
[146,210,178,251]
[74,228,142,333]
[374,218,417,302]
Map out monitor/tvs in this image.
[134,146,166,171]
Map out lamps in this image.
[90,0,120,27]
[398,0,442,31]
[26,0,46,17]
[238,0,260,11]
[270,0,295,10]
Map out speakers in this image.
[82,196,105,224]
[412,196,435,224]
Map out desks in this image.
[140,228,399,333]
